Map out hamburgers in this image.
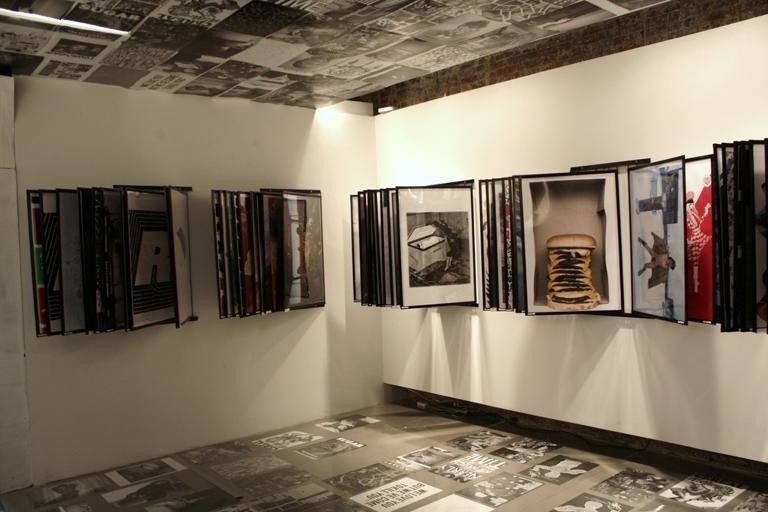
[545,233,603,311]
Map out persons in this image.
[636,236,675,276]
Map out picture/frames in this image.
[26,185,198,336]
[209,186,325,320]
